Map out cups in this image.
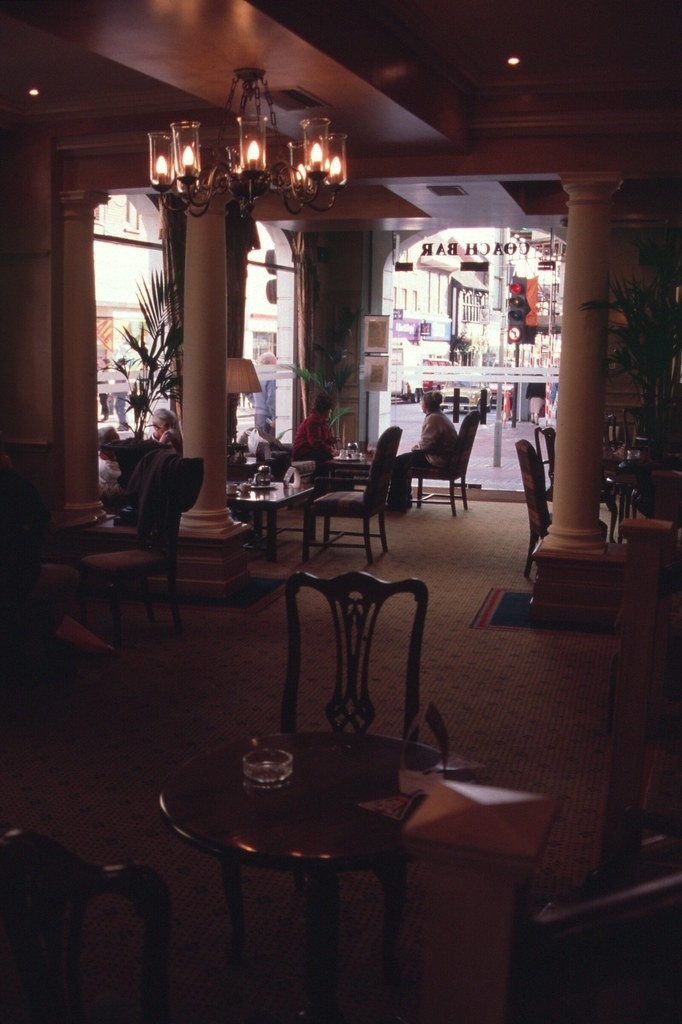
[237,483,251,496]
[228,486,237,494]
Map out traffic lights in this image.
[509,281,525,320]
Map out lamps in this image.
[225,358,263,463]
[146,66,349,219]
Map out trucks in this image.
[390,359,459,402]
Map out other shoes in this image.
[385,502,412,512]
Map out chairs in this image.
[222,570,429,990]
[0,447,185,700]
[510,405,649,582]
[400,411,481,517]
[302,424,404,565]
[0,827,172,1024]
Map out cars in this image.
[439,379,492,416]
[490,382,516,409]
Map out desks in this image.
[324,453,372,493]
[156,730,481,1024]
[223,479,318,563]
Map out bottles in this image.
[258,465,271,485]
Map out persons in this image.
[292,395,342,473]
[98,358,129,431]
[98,427,125,498]
[525,374,559,426]
[153,409,183,459]
[386,391,458,513]
[244,350,278,439]
